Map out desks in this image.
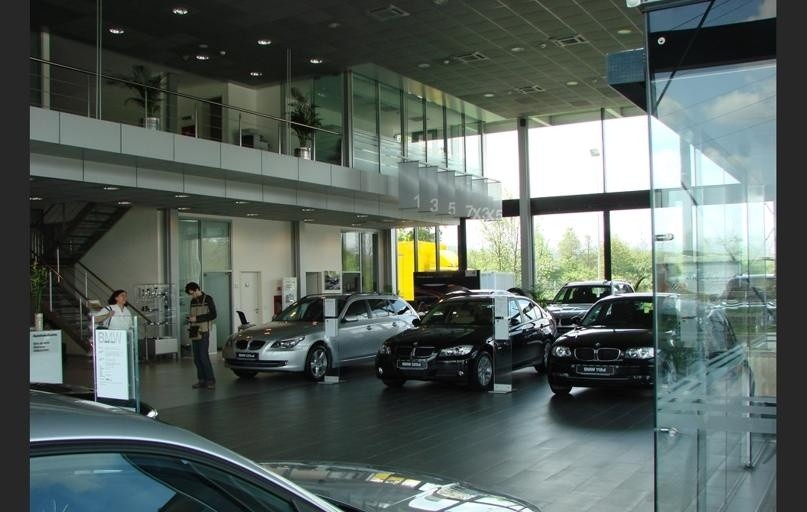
[143,338,178,363]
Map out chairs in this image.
[450,311,475,324]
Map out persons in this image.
[183,282,216,391]
[92,290,132,331]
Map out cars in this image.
[222,292,421,381]
[30,380,541,511]
[544,279,635,334]
[547,292,681,393]
[719,273,777,330]
[374,288,558,389]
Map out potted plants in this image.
[107,66,170,130]
[285,88,323,159]
[29,262,48,331]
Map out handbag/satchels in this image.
[189,329,203,342]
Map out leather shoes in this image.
[192,380,216,390]
